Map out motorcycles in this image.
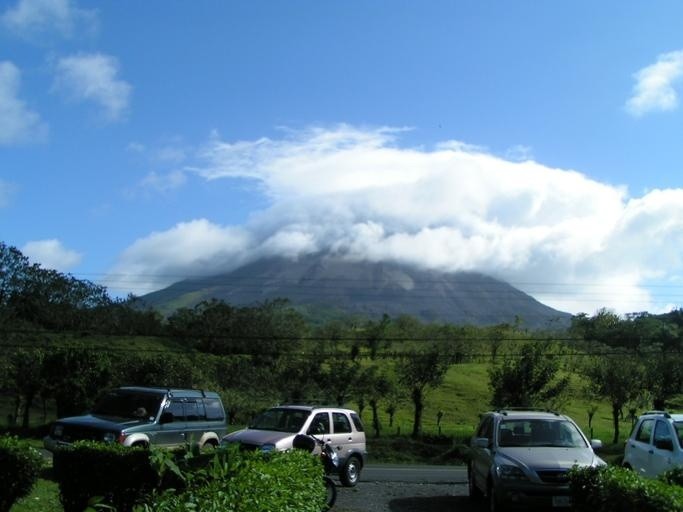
[292,420,340,511]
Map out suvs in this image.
[620,411,682,483]
[43,381,225,463]
[466,408,609,510]
[223,399,369,484]
